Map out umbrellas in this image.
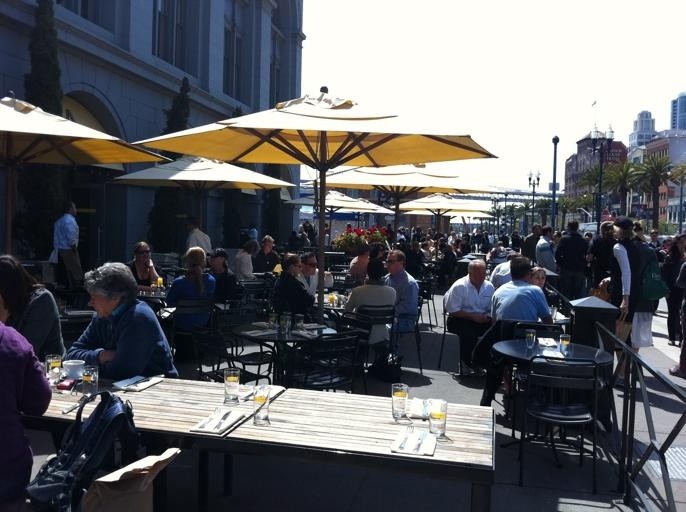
[111,156,297,236]
[283,192,497,247]
[129,86,499,329]
[0,89,174,259]
[303,160,501,250]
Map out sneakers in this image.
[668,340,681,347]
[669,365,686,378]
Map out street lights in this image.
[551,134,560,227]
[527,168,542,226]
[466,216,471,232]
[588,117,616,236]
[489,194,500,235]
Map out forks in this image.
[399,425,415,450]
[197,408,221,428]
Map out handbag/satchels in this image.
[615,319,632,341]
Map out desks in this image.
[223,387,495,510]
[19,366,286,510]
[492,337,614,463]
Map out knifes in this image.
[214,410,232,431]
[242,386,264,399]
[412,431,428,452]
[63,395,96,413]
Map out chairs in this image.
[491,319,563,339]
[519,355,599,494]
[137,272,285,334]
[418,281,438,331]
[327,264,355,294]
[191,293,424,395]
[439,297,462,374]
[45,271,89,309]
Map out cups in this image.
[224,368,241,405]
[329,291,352,306]
[549,307,558,320]
[392,383,409,419]
[139,281,171,295]
[253,385,269,421]
[525,329,537,348]
[39,353,100,400]
[427,398,449,437]
[560,334,571,352]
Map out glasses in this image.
[306,263,318,267]
[651,231,659,235]
[136,249,152,255]
[385,259,398,265]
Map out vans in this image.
[578,221,602,239]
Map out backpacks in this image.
[635,246,672,303]
[22,390,148,512]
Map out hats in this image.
[614,215,635,230]
[206,247,227,257]
